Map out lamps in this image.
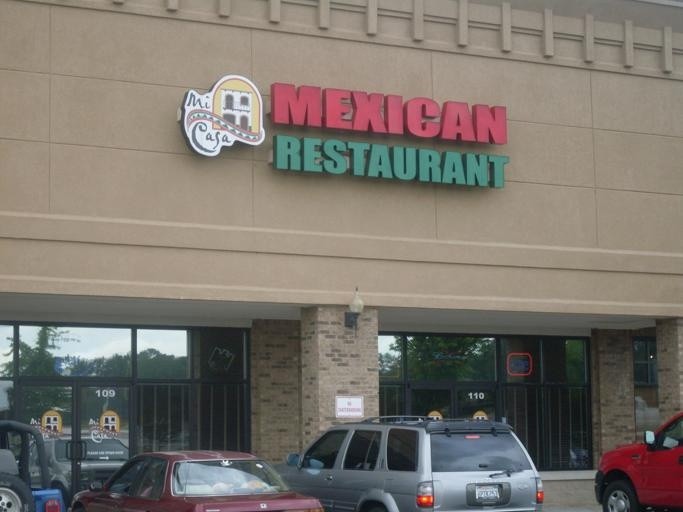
[345,286,363,338]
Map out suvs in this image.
[0,418,66,512]
[20,436,133,500]
[264,415,546,512]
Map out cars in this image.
[589,409,683,512]
[64,446,325,512]
